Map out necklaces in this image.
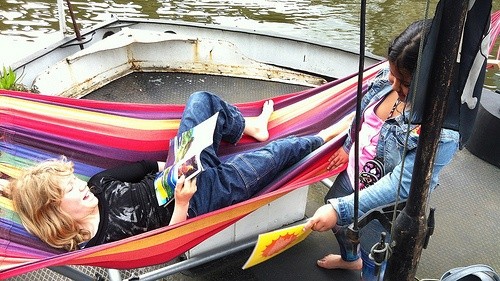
[386,98,402,119]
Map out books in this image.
[154,111,219,210]
[242,221,312,270]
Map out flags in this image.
[459,0,493,151]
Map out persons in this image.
[11,91,356,253]
[302,18,459,281]
